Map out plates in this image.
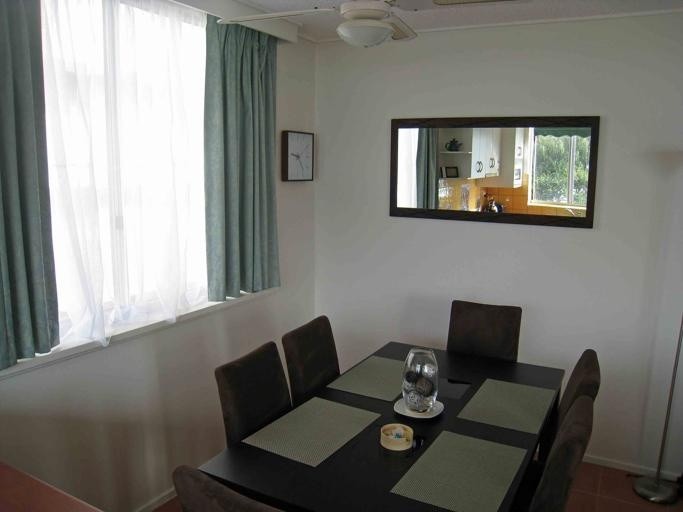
[392,398,445,419]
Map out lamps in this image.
[337,0,392,51]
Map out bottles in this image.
[401,347,438,411]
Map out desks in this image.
[195,339,566,510]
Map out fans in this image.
[215,1,507,49]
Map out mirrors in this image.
[388,114,601,230]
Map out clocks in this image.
[280,130,315,183]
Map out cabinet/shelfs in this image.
[471,129,485,179]
[485,128,501,177]
[476,127,523,188]
[438,128,472,179]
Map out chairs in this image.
[556,347,600,425]
[281,314,340,407]
[445,300,523,362]
[169,464,280,510]
[528,394,595,510]
[213,338,291,447]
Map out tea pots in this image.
[444,137,463,151]
